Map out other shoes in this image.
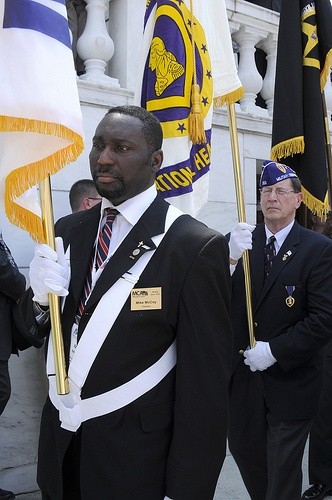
[0,488,15,500]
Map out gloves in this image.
[229,223,256,260]
[243,341,277,372]
[29,237,69,306]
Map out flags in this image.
[270,0,332,229]
[139,0,257,352]
[1,2,83,397]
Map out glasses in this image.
[259,189,298,197]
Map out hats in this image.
[260,160,298,187]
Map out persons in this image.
[1,104,332,499]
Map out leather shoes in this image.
[302,483,332,500]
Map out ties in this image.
[265,236,277,282]
[74,208,120,326]
